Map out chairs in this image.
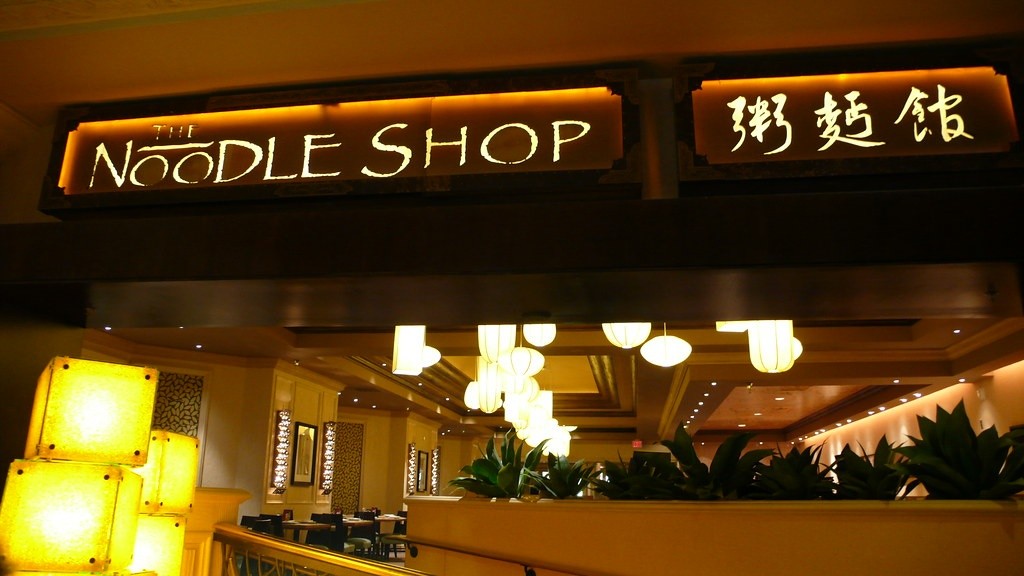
[241,508,406,558]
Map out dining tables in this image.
[375,516,406,536]
[342,518,374,538]
[282,521,330,545]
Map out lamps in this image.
[392,320,803,458]
